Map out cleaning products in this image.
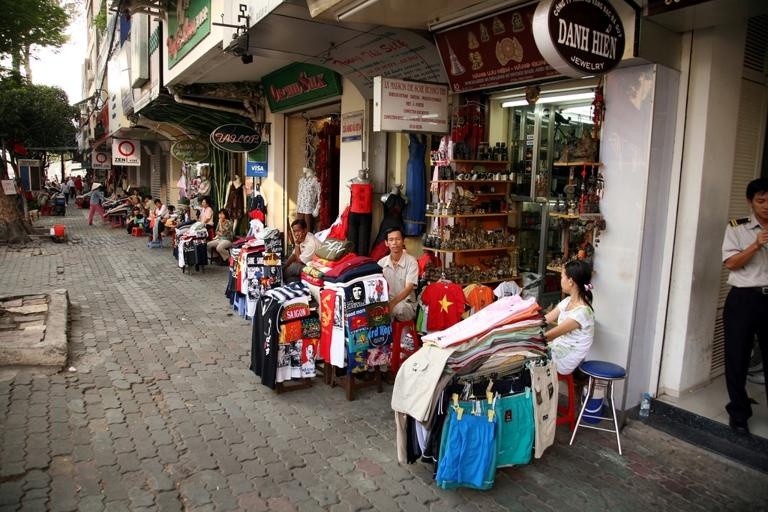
[639,393,652,416]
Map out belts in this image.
[741,286,767,295]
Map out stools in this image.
[554,359,630,456]
[131,227,142,238]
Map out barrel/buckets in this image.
[54,225,64,236]
[580,384,605,424]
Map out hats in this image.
[193,178,212,197]
[249,209,264,222]
[91,183,102,191]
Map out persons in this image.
[544,259,596,376]
[296,166,322,233]
[44,173,176,242]
[278,339,316,377]
[345,169,376,256]
[207,208,234,264]
[351,282,365,302]
[282,218,323,284]
[375,279,384,301]
[223,174,248,234]
[198,198,215,240]
[376,225,420,322]
[721,177,768,437]
[248,257,281,300]
[379,183,409,241]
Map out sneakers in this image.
[728,417,749,437]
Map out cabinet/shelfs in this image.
[545,159,602,273]
[419,157,522,286]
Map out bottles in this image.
[639,391,651,417]
